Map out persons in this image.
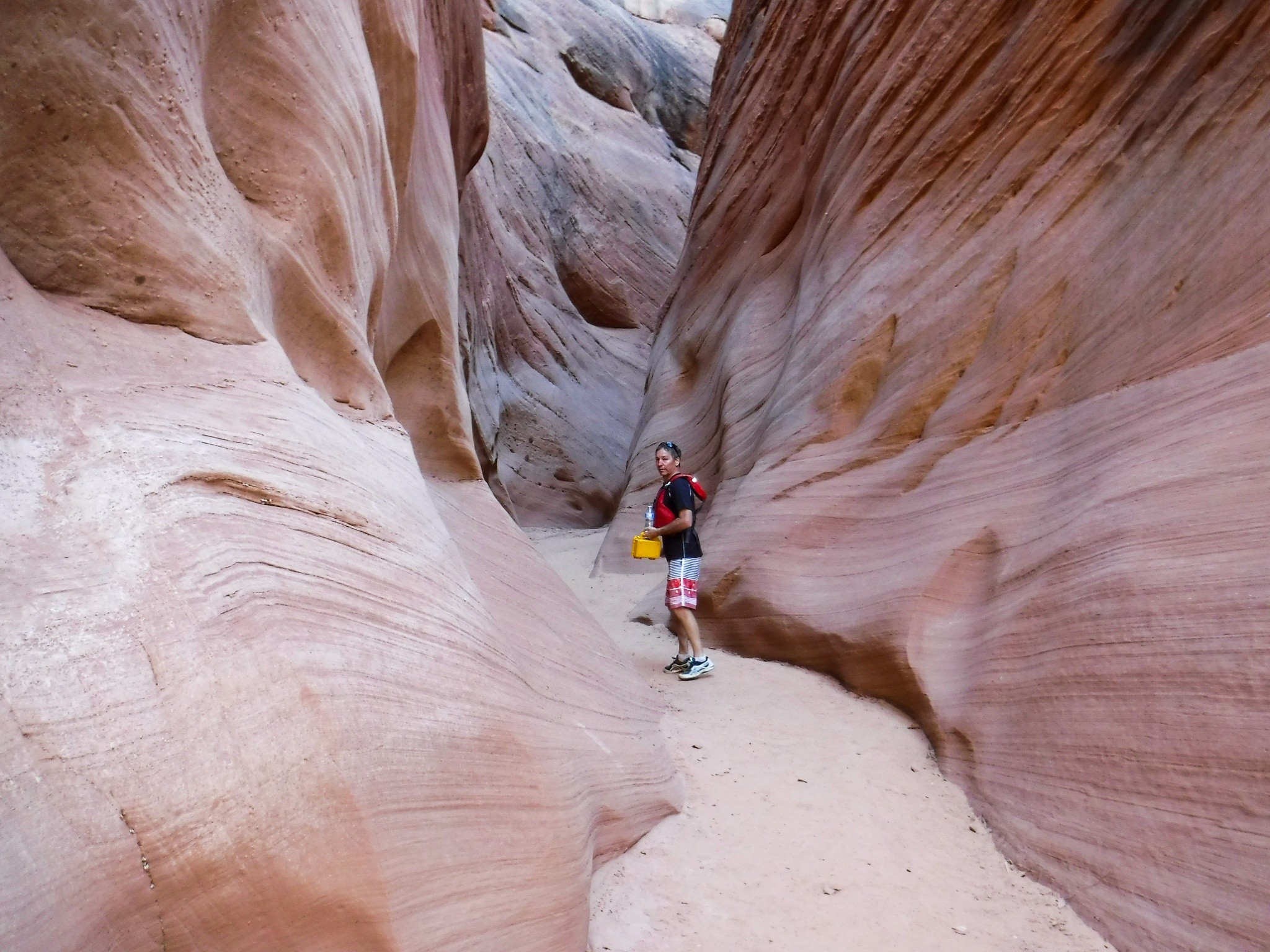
[643,440,715,680]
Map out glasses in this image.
[666,441,679,458]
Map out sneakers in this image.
[678,656,714,679]
[664,654,693,673]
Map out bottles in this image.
[644,506,655,528]
